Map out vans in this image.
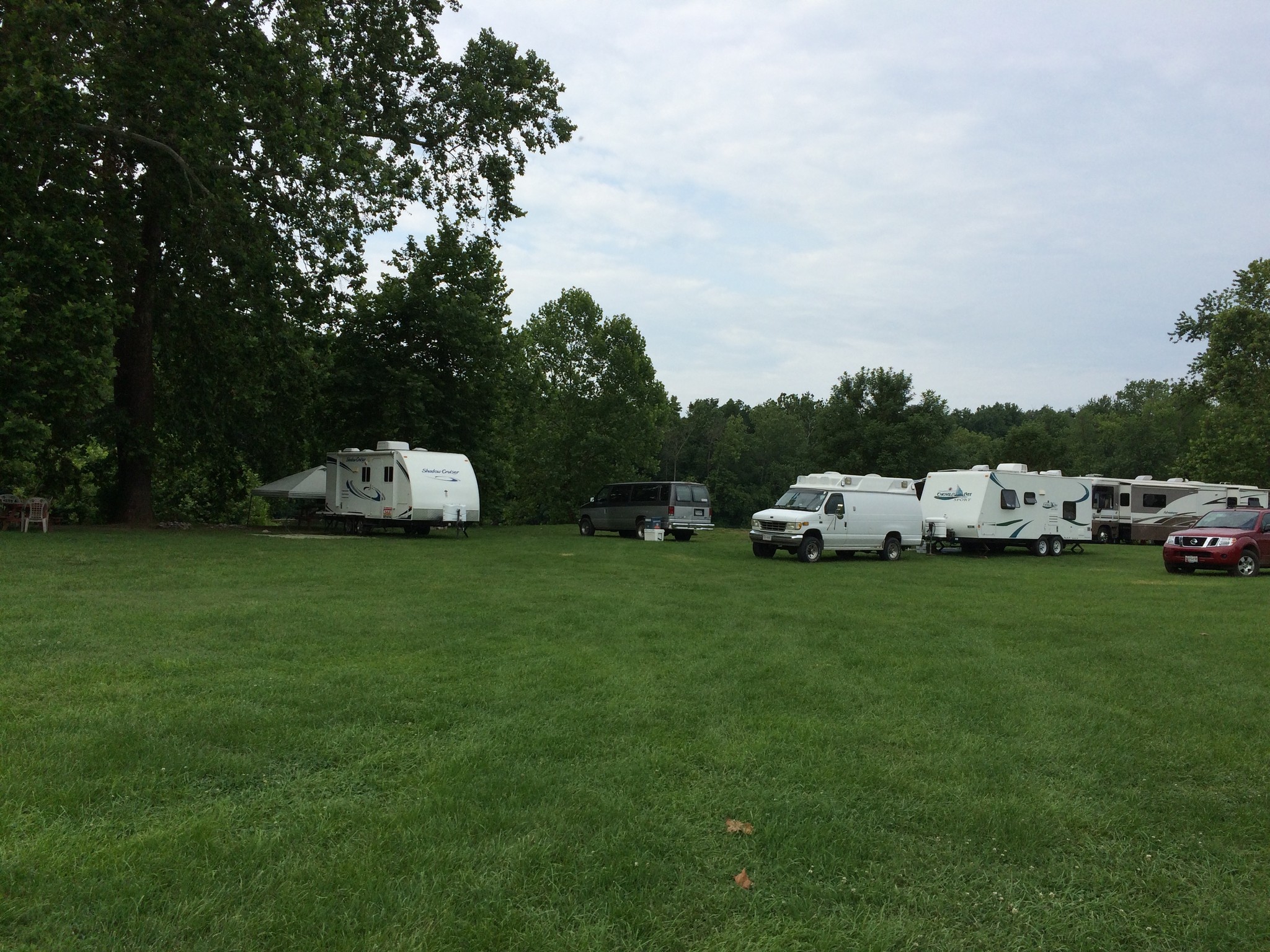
[577,481,716,542]
[749,471,924,564]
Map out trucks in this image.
[1085,473,1270,545]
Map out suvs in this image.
[1162,505,1270,578]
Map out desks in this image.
[316,511,365,536]
[291,515,324,533]
[3,502,47,531]
[274,519,298,534]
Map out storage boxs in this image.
[643,528,665,541]
[644,516,663,529]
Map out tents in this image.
[244,465,327,528]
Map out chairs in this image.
[0,494,53,533]
